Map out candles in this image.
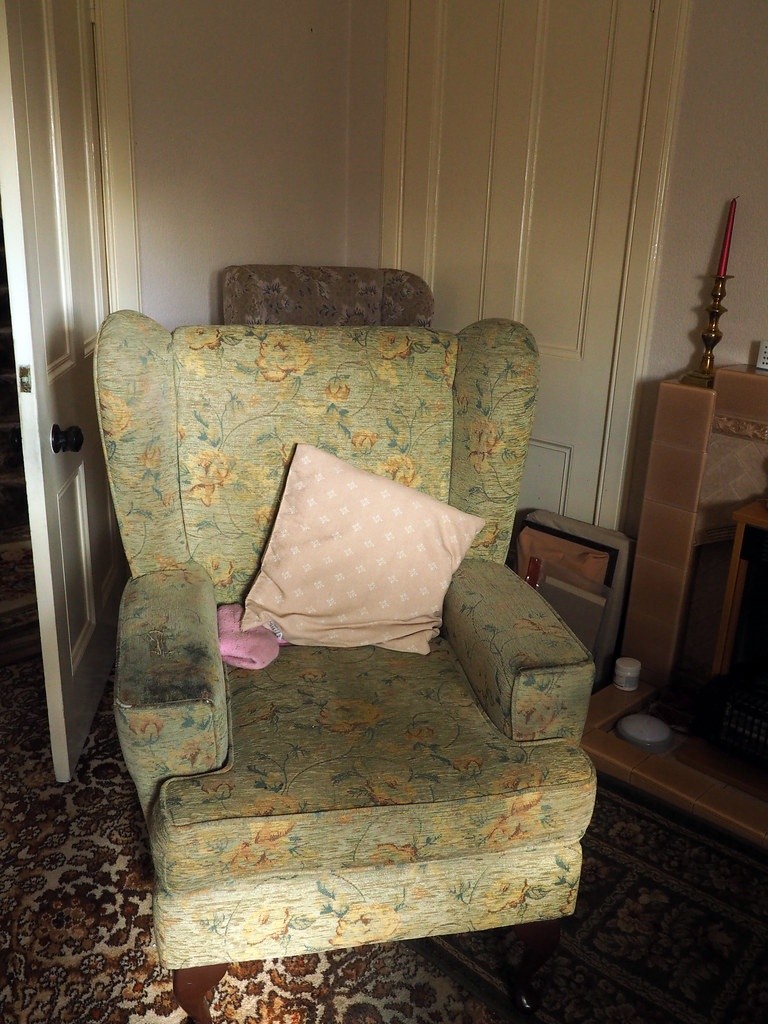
[718,194,740,276]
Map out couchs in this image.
[219,264,435,330]
[93,308,596,972]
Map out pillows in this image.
[240,444,488,658]
